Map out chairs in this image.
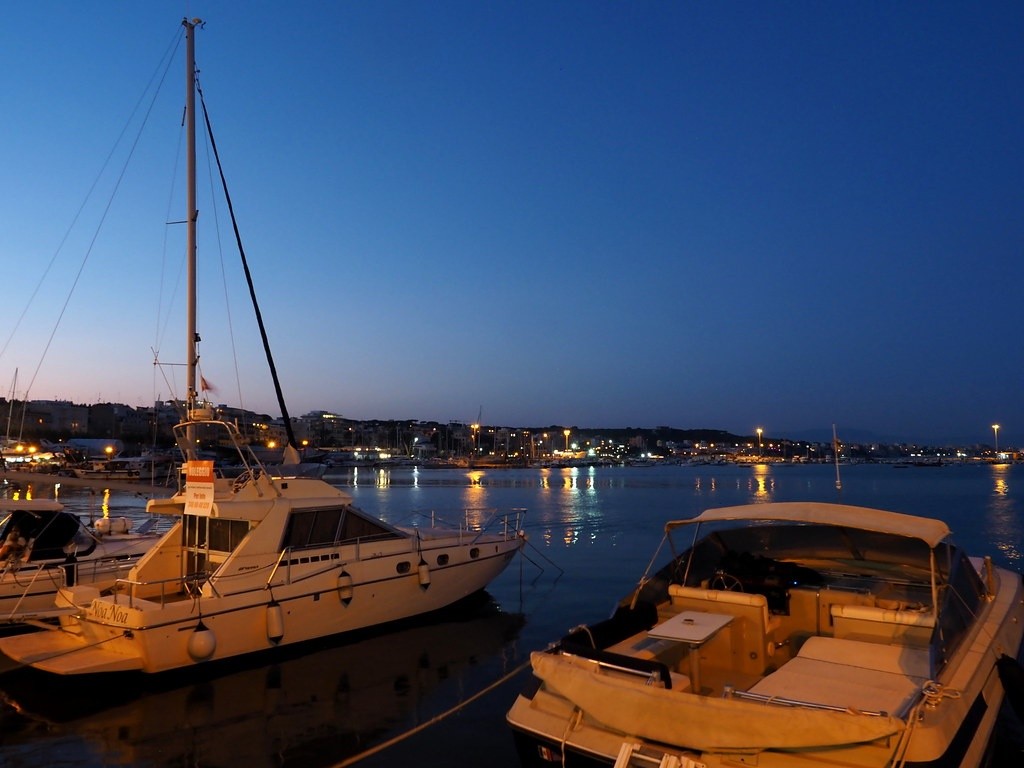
[179,561,215,602]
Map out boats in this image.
[893,465,908,468]
[837,461,850,465]
[507,501,1023,767]
[218,462,327,478]
[769,462,796,467]
[73,458,177,481]
[737,463,753,467]
[0,494,169,624]
[914,459,939,466]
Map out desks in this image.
[648,610,734,694]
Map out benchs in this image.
[831,605,936,650]
[561,601,658,659]
[668,583,781,634]
[563,645,690,692]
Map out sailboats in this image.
[0,18,530,716]
[832,424,843,490]
[324,422,728,470]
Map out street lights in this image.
[563,430,570,452]
[757,429,762,456]
[992,425,1000,451]
[471,424,478,455]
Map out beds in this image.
[744,636,932,718]
